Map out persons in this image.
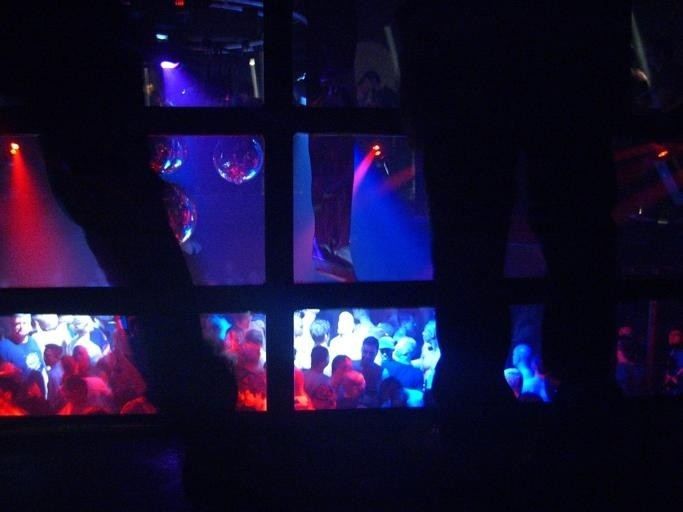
[2,3,681,408]
